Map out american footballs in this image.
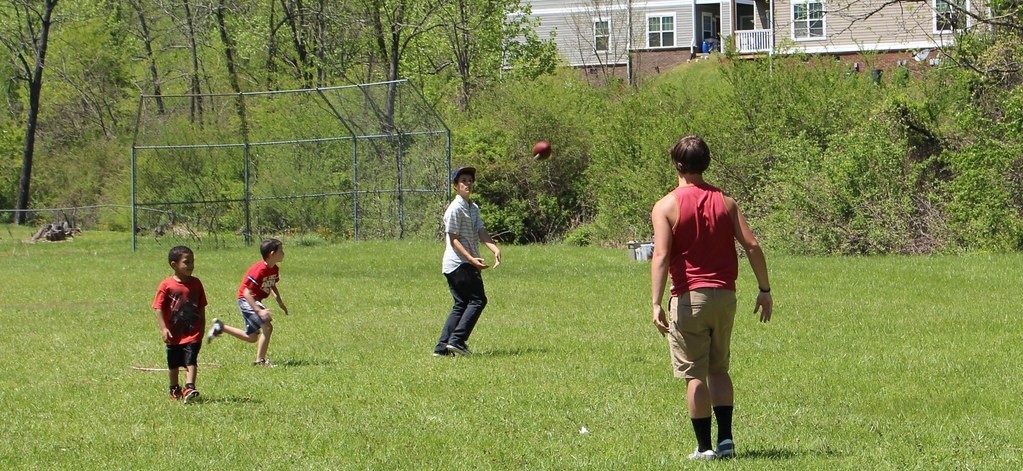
[531,141,552,161]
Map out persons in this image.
[433,166,502,358]
[650,135,774,462]
[151,245,208,404]
[206,237,289,368]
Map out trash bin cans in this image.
[625,240,655,261]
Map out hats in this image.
[452,166,476,181]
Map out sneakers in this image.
[447,336,472,356]
[716,439,735,459]
[434,347,455,357]
[206,319,224,343]
[687,446,717,460]
[254,360,277,369]
[170,384,184,400]
[182,383,199,403]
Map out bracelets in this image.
[758,286,771,293]
